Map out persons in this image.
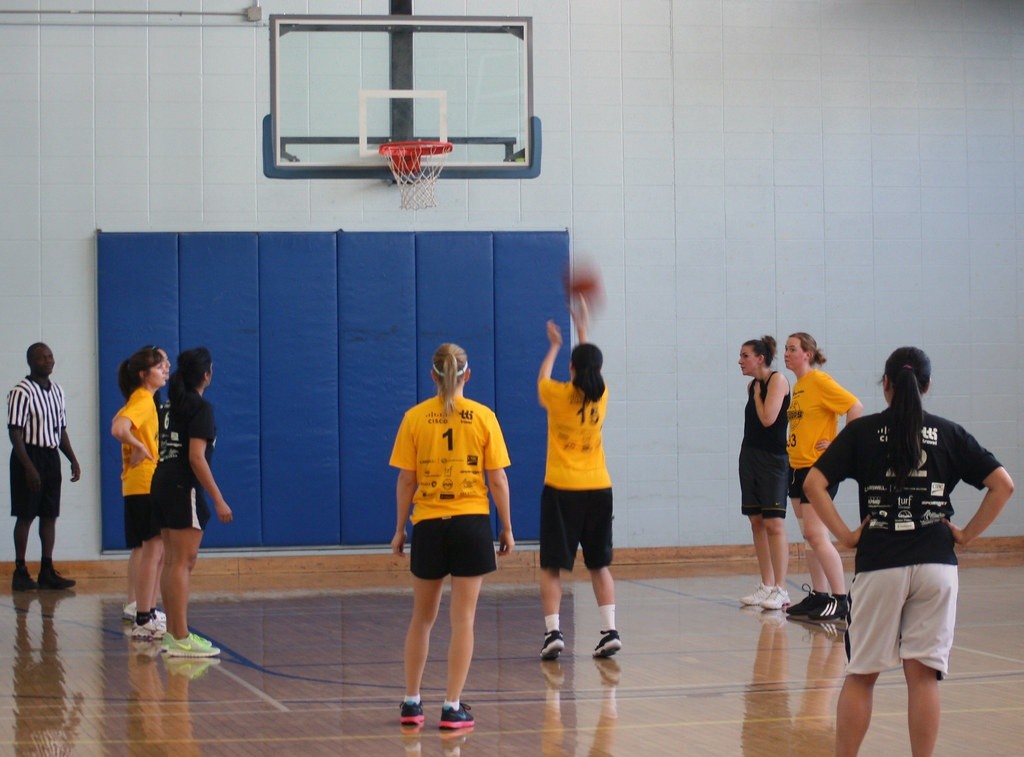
[783,331,863,624]
[147,347,233,658]
[737,336,792,611]
[388,343,515,729]
[5,342,80,591]
[536,296,622,661]
[801,345,1014,756]
[109,345,171,640]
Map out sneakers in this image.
[593,628,621,658]
[400,721,424,747]
[438,702,474,729]
[161,652,221,680]
[399,700,425,723]
[785,590,828,614]
[593,656,620,686]
[437,726,474,748]
[12,566,40,590]
[761,584,791,609]
[786,615,845,643]
[12,588,76,607]
[131,619,169,640]
[162,631,220,657]
[38,570,76,590]
[539,631,566,661]
[745,607,789,628]
[809,594,848,620]
[122,601,167,623]
[740,582,775,606]
[122,619,133,636]
[540,660,565,690]
[126,637,164,658]
